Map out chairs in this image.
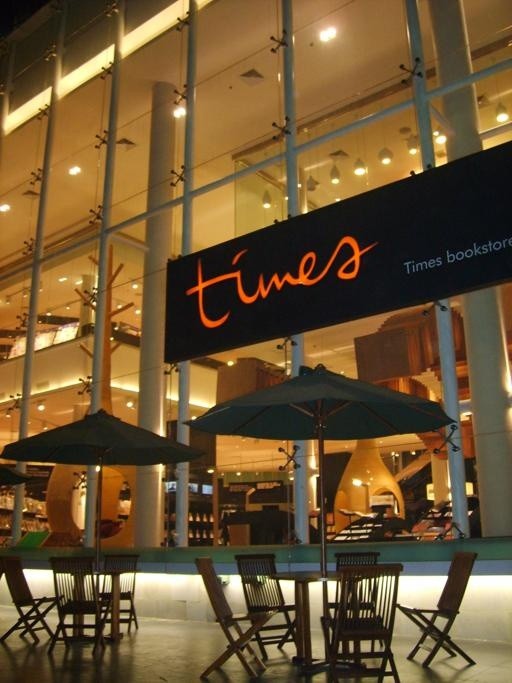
[1,555,145,655]
[192,546,477,680]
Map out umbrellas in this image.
[182,363,456,617]
[2,407,207,571]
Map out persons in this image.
[382,505,401,539]
